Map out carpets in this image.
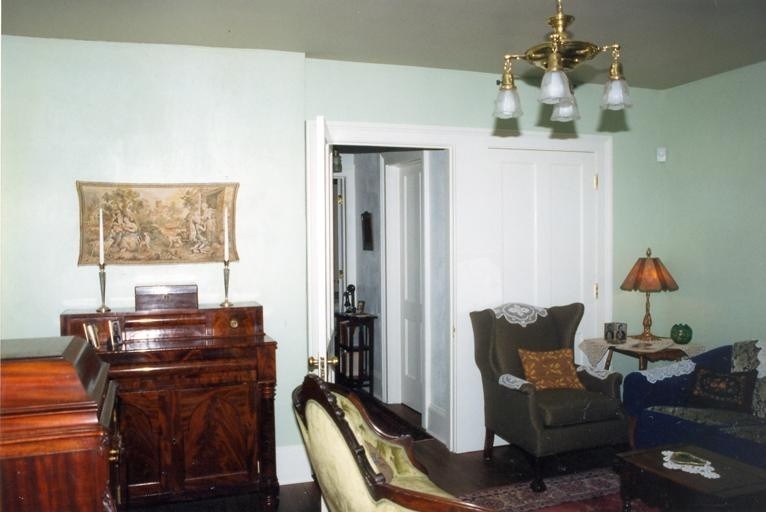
[456,466,661,512]
[336,386,434,443]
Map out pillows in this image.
[688,367,755,414]
[518,348,584,391]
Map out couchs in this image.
[470,303,630,494]
[292,375,494,512]
[625,340,766,471]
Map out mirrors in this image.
[333,176,346,312]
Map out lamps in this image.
[620,248,679,341]
[493,0,633,123]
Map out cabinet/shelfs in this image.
[335,312,378,396]
[60,302,280,512]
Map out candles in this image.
[100,208,104,265]
[224,206,229,261]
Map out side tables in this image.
[579,338,706,372]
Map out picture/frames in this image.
[75,182,238,264]
[358,301,365,312]
